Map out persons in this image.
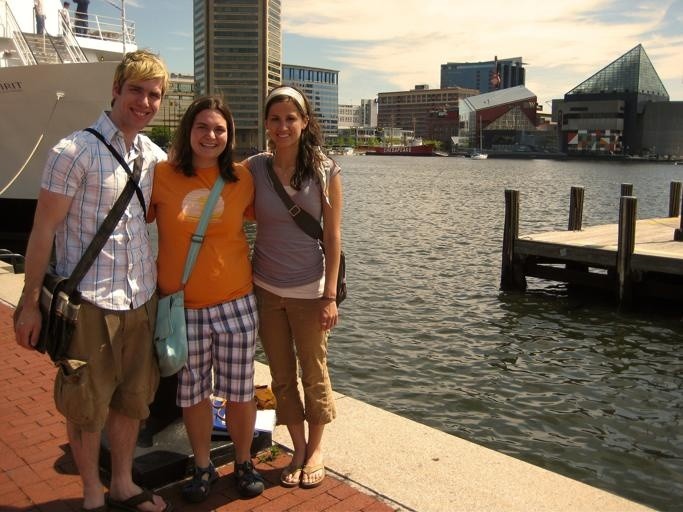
[238,86,343,488]
[14,49,169,511]
[149,98,264,504]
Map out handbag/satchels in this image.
[34,273,83,363]
[154,290,187,378]
[337,250,346,308]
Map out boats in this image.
[372,142,435,156]
[0,0,141,232]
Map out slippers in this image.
[281,458,326,487]
[81,485,173,512]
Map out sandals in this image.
[182,461,219,503]
[234,458,264,497]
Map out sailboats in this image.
[469,113,490,161]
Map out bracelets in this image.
[322,297,336,301]
